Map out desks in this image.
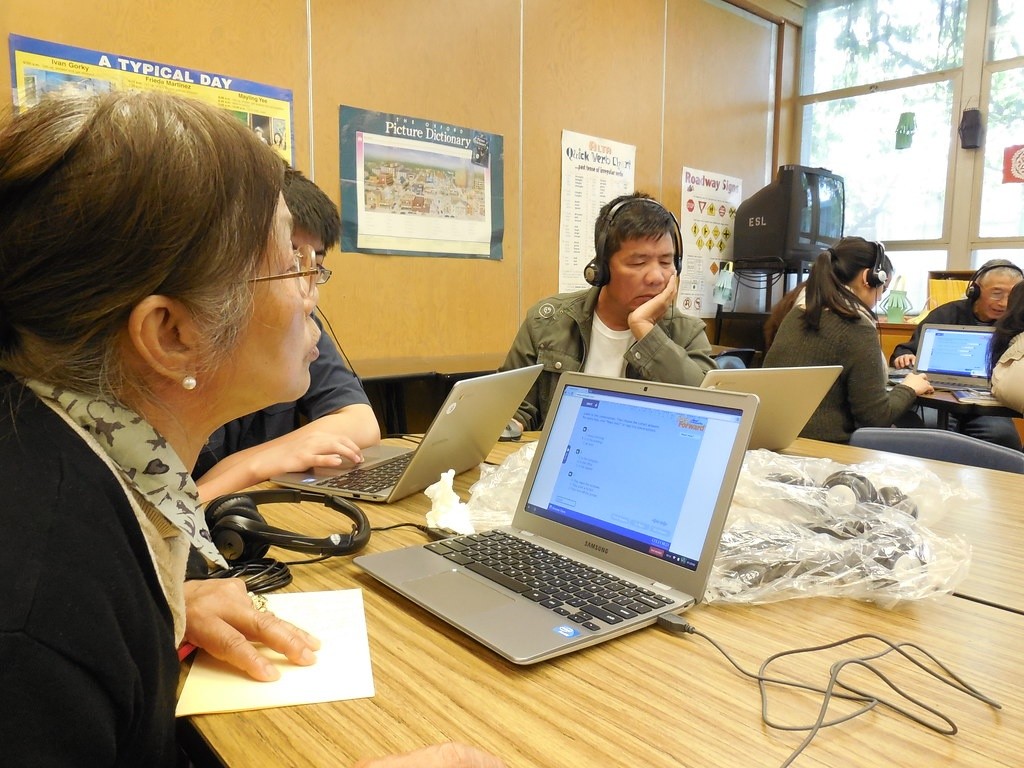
[886,366,1023,430]
[177,431,1024,768]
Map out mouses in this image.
[499,418,523,441]
[904,360,914,370]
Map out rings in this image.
[248,593,267,614]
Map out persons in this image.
[759,237,934,445]
[0,91,509,768]
[889,260,1024,453]
[496,194,721,438]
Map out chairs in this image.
[707,343,766,372]
[849,427,1024,475]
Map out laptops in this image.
[889,324,996,393]
[699,365,844,450]
[268,364,545,505]
[354,371,760,667]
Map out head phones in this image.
[966,264,1023,303]
[866,241,887,289]
[583,197,683,288]
[204,490,371,567]
[765,472,917,564]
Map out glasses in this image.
[316,267,333,285]
[979,282,1010,301]
[157,243,316,299]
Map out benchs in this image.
[349,372,509,437]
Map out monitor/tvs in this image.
[733,165,845,262]
